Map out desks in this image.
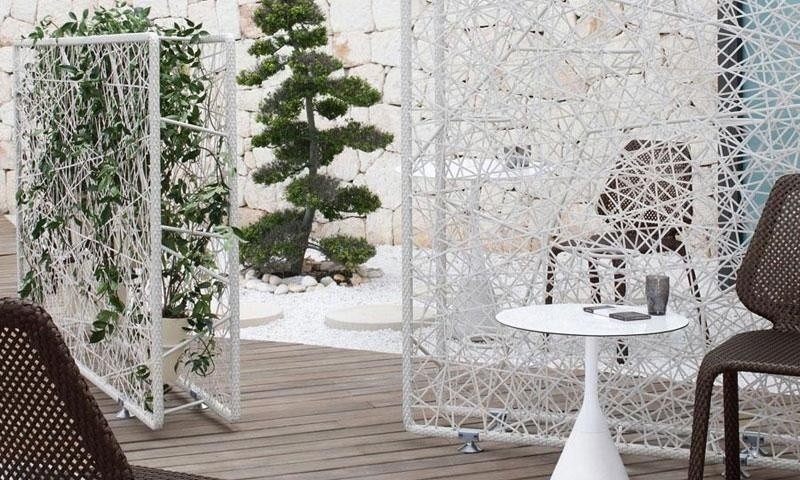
[496,305,687,480]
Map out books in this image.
[583,305,651,321]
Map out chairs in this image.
[686,172,800,480]
[1,296,215,479]
[539,138,715,364]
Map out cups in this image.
[646,276,669,315]
